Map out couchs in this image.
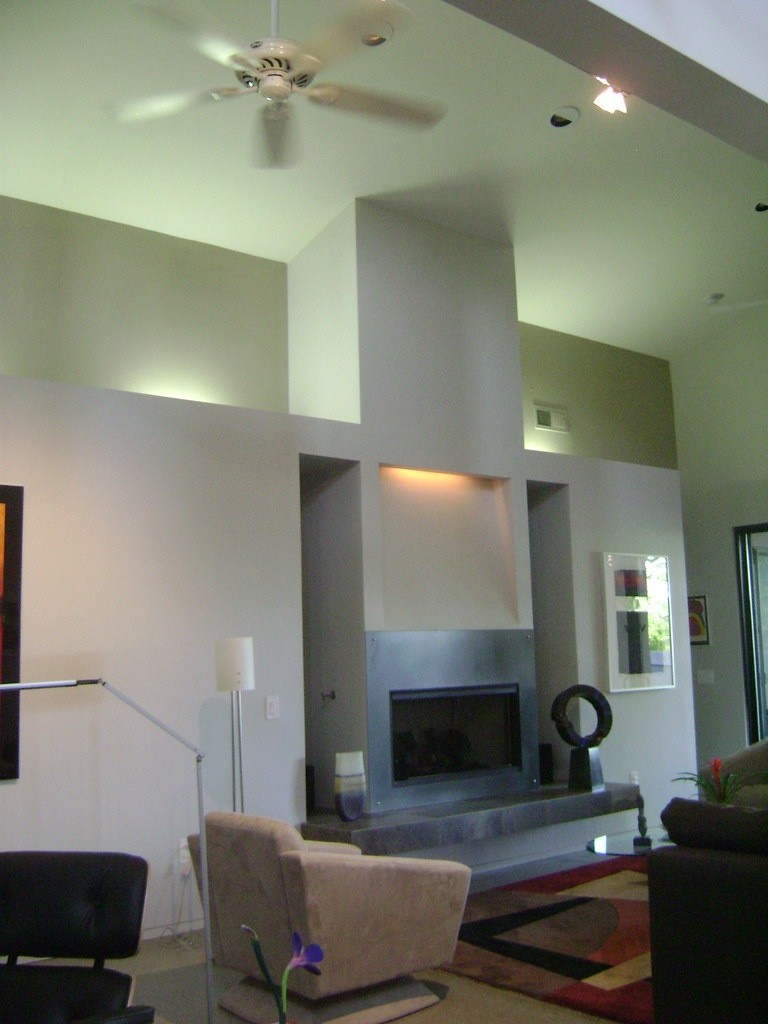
[187,808,469,1022]
[699,735,767,808]
[647,795,768,1023]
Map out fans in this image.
[116,0,446,164]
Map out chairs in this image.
[0,852,155,1024]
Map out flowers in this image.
[236,915,327,1024]
[673,757,767,803]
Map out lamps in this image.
[218,637,256,814]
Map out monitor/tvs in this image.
[390,683,523,788]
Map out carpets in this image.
[437,849,655,1024]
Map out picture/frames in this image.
[688,594,710,646]
